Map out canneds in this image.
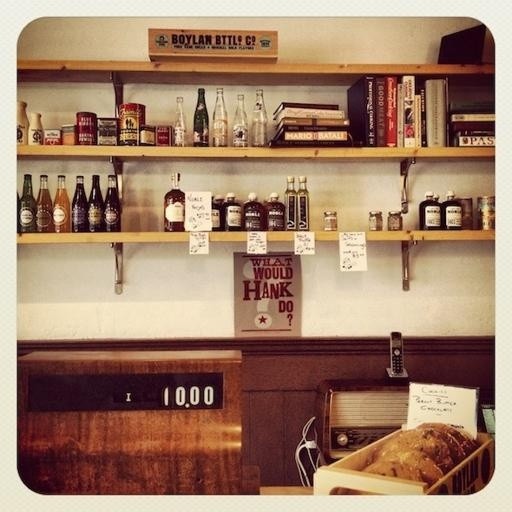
[455,197,473,229]
[324,211,337,230]
[369,212,382,230]
[388,211,402,230]
[43,103,175,146]
[478,195,495,230]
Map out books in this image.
[267,73,495,147]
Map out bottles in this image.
[417,189,463,231]
[164,172,285,232]
[19,172,123,233]
[171,87,268,147]
[285,176,309,229]
[17,100,45,146]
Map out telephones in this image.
[386,331,409,380]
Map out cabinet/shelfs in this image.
[17,141,495,244]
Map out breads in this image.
[336,423,481,495]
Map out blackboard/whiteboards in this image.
[407,381,481,442]
